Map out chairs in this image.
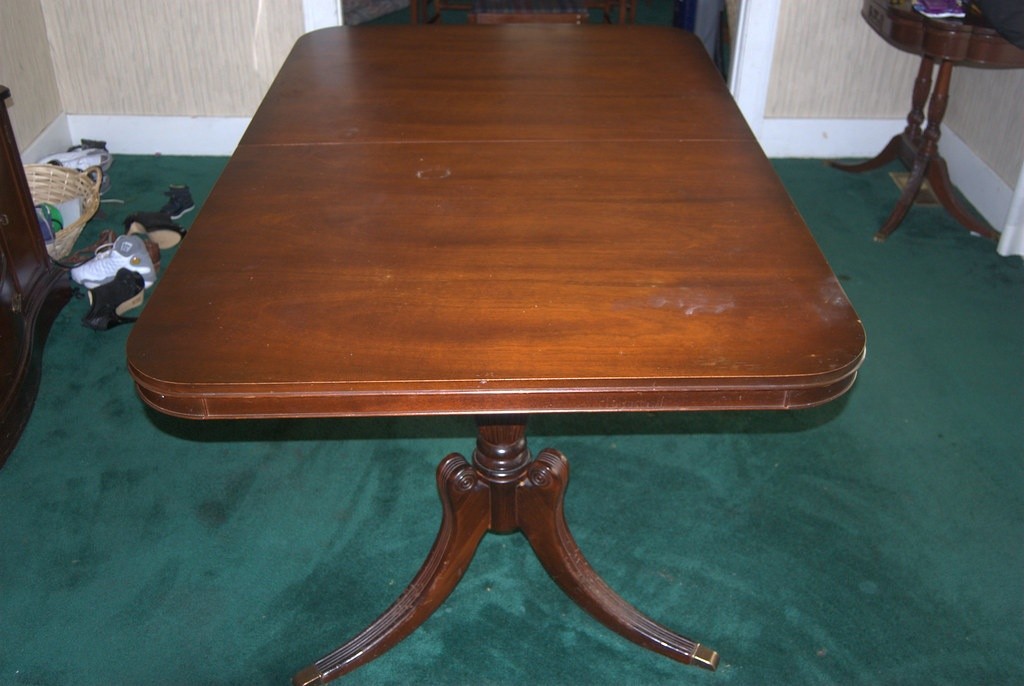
[410,0,636,25]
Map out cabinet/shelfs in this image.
[0,85,72,469]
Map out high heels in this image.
[79,267,147,331]
[122,214,187,251]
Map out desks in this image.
[822,0,1024,244]
[125,22,867,686]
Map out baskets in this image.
[23,162,104,261]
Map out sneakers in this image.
[36,147,114,176]
[160,185,196,220]
[67,233,158,289]
